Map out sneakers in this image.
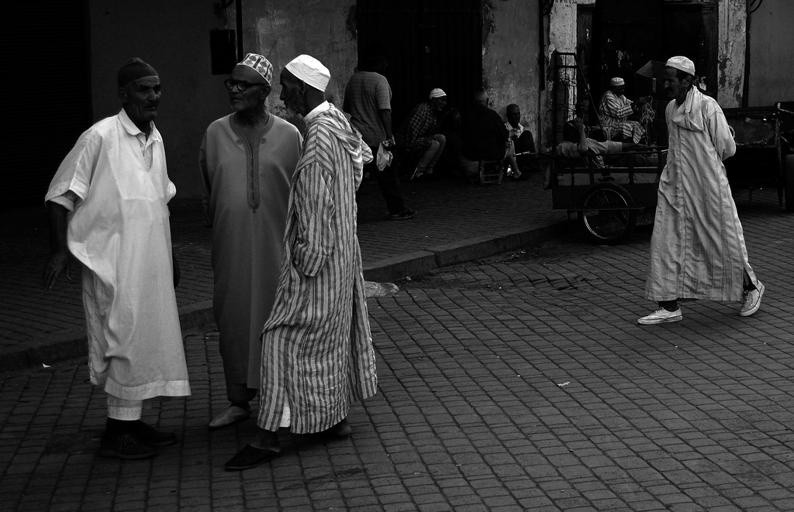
[735,278,767,319]
[633,307,686,326]
[130,422,177,446]
[387,205,419,221]
[96,430,159,462]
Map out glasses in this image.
[222,78,267,92]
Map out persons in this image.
[38,57,193,464]
[195,53,307,434]
[636,56,766,326]
[220,51,380,471]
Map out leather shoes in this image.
[206,403,252,432]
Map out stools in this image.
[477,158,508,186]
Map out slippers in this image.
[222,443,284,471]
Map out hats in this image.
[232,52,275,91]
[280,53,332,94]
[116,55,161,88]
[608,77,625,87]
[426,87,448,101]
[661,55,697,78]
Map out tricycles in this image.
[721,100,793,210]
[551,142,670,244]
